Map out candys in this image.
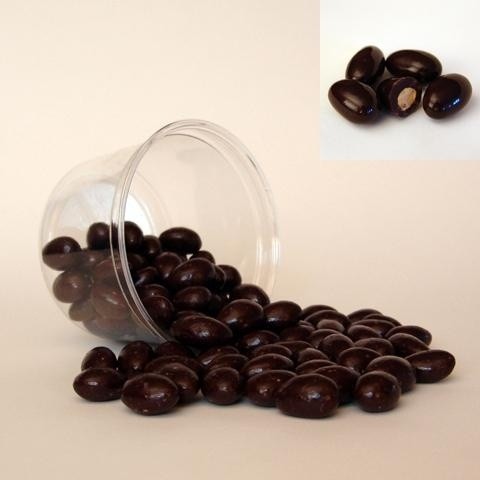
[326,46,471,124]
[43,221,456,420]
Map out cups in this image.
[35,116,280,354]
